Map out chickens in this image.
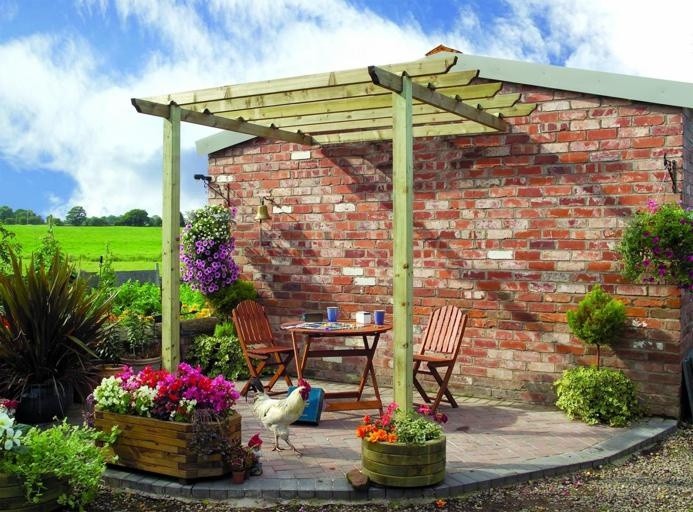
[247,376,312,458]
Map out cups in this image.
[326,306,337,321]
[373,309,385,325]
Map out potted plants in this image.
[0,416,121,512]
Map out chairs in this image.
[408,304,471,415]
[227,298,297,400]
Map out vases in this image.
[355,435,447,489]
[1,246,179,425]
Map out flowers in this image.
[172,204,243,295]
[0,399,23,452]
[612,196,691,294]
[354,402,449,451]
[82,360,262,484]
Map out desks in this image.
[280,319,387,422]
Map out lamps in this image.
[193,174,230,208]
